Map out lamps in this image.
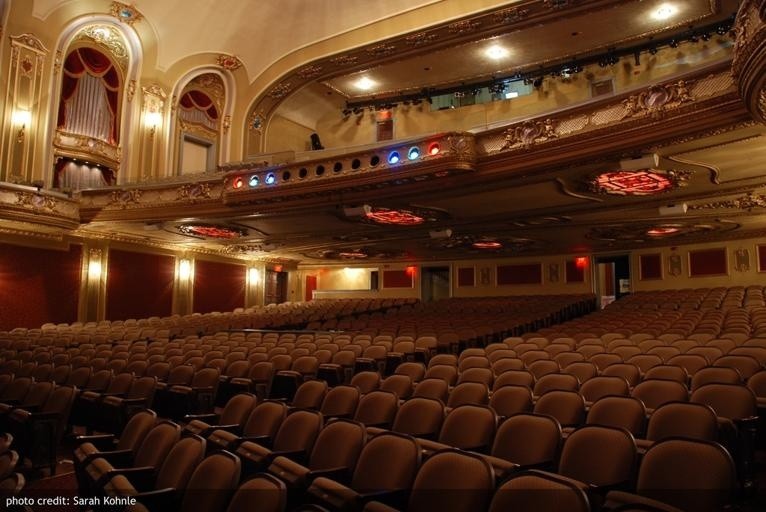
[222,114,231,134]
[428,227,451,240]
[343,203,370,218]
[141,221,164,234]
[337,20,734,116]
[618,154,659,172]
[145,114,163,140]
[13,110,33,144]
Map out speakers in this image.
[344,204,372,217]
[429,229,452,239]
[619,152,660,170]
[261,244,276,250]
